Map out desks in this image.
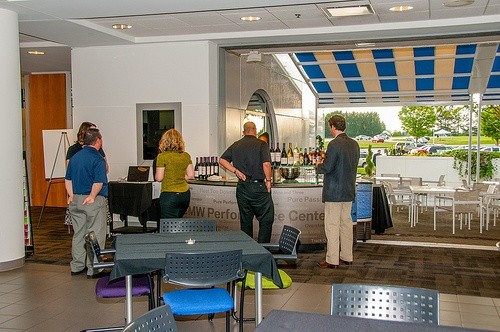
[372,177,411,212]
[409,186,469,230]
[108,230,283,327]
[252,308,500,332]
[108,181,162,234]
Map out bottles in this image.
[280,142,286,165]
[299,147,320,166]
[275,143,281,166]
[270,142,275,165]
[194,156,219,180]
[287,142,293,166]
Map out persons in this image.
[156,128,194,218]
[315,114,361,268]
[65,122,112,278]
[219,122,275,248]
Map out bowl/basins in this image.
[279,168,300,180]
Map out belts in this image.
[242,178,263,183]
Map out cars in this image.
[356,133,389,143]
[396,136,500,155]
[357,151,369,168]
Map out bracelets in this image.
[267,180,271,181]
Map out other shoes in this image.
[340,259,353,265]
[87,271,110,279]
[71,266,88,276]
[316,261,339,268]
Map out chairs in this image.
[362,173,500,235]
[83,218,301,332]
[329,284,440,325]
